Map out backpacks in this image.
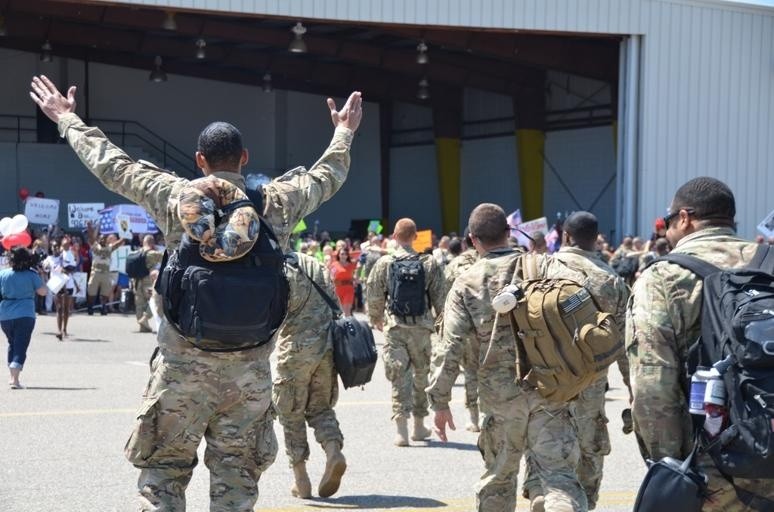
[153,187,291,354]
[632,241,773,512]
[125,247,155,292]
[491,250,627,407]
[384,251,431,319]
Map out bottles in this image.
[687,363,725,417]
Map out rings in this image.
[40,93,46,99]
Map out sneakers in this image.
[527,484,546,511]
[136,316,157,333]
[8,383,27,390]
[56,329,69,340]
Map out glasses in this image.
[470,224,511,245]
[663,206,697,231]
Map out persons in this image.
[0,246,50,390]
[367,216,445,448]
[272,249,346,501]
[623,176,773,512]
[550,209,630,512]
[423,203,633,512]
[26,72,363,512]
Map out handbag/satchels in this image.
[331,309,378,391]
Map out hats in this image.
[9,246,39,262]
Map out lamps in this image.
[145,51,169,84]
[285,21,307,54]
[259,70,275,94]
[34,27,51,60]
[413,39,429,65]
[194,35,208,60]
[417,76,435,99]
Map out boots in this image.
[318,439,348,499]
[289,460,312,500]
[465,406,481,432]
[410,413,433,442]
[391,416,410,447]
